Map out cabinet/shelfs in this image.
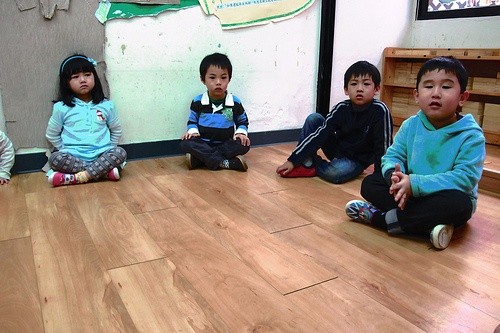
[379,46,500,146]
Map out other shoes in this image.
[430,225,454,249]
[345,200,381,226]
[48,172,77,186]
[105,167,121,180]
[186,153,205,169]
[228,155,248,171]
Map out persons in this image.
[46,54,126,187]
[345,58,486,250]
[0,130,15,185]
[276,60,393,185]
[182,52,250,171]
